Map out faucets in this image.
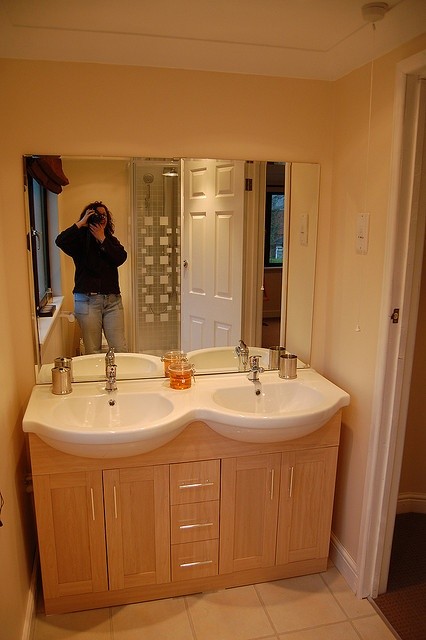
[106,365,117,390]
[249,356,264,379]
[106,348,115,365]
[236,340,249,367]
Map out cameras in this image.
[86,210,101,225]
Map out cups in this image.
[268,346,286,369]
[278,354,297,380]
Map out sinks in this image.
[187,347,273,369]
[71,353,161,379]
[49,391,174,433]
[211,381,328,417]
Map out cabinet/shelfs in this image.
[27,408,344,616]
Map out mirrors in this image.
[23,153,322,386]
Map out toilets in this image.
[79,331,111,354]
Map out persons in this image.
[54,200,128,355]
